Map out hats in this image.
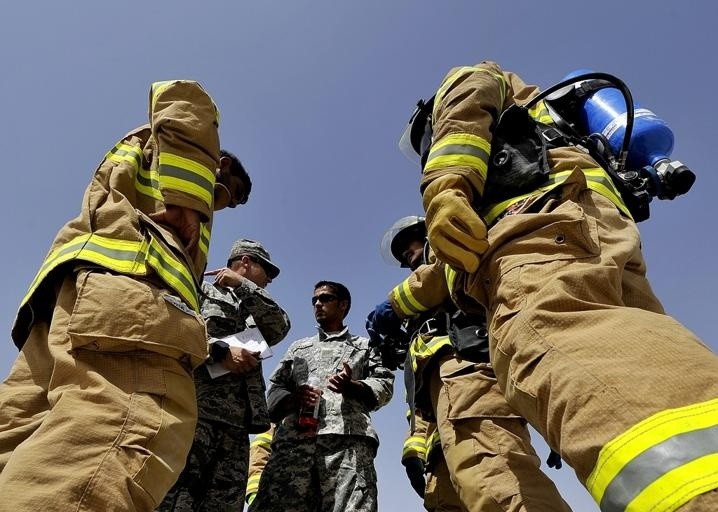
[227,239,279,279]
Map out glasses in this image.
[311,294,338,306]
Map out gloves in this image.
[366,300,408,363]
[423,173,489,273]
[403,456,427,499]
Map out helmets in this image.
[380,216,425,267]
[398,93,435,169]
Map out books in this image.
[204,314,274,379]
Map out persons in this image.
[247,281,397,511]
[0,79,252,512]
[396,59,717,512]
[155,239,291,511]
[368,213,573,511]
[245,415,286,504]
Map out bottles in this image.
[298,370,323,427]
